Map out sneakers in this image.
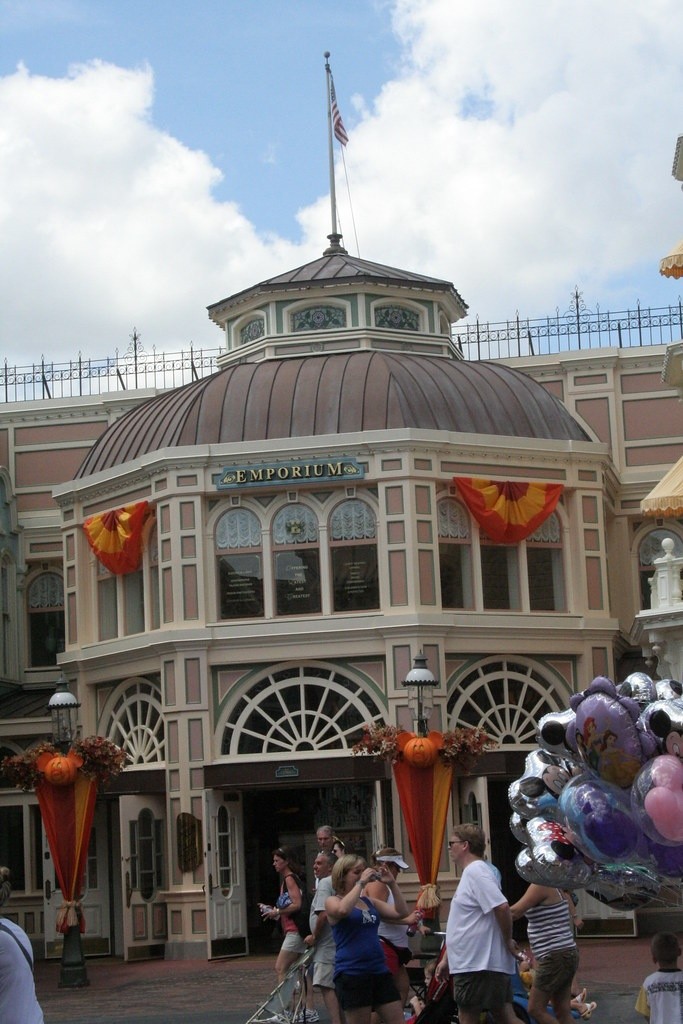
[291,1009,320,1023]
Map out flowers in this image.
[1,742,59,793]
[351,721,403,767]
[439,727,501,777]
[75,735,133,792]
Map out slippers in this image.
[581,1001,597,1020]
[576,988,587,1002]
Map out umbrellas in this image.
[658,242,683,281]
[640,457,683,519]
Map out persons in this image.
[257,823,598,1024]
[634,932,683,1024]
[0,866,45,1024]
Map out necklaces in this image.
[336,895,343,900]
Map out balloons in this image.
[508,673,683,911]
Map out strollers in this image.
[426,930,580,1024]
[402,944,453,1024]
[246,945,317,1024]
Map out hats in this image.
[377,856,410,868]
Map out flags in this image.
[330,73,351,146]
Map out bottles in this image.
[259,903,280,920]
[406,912,420,937]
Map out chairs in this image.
[406,967,427,1007]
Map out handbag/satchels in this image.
[284,874,313,939]
[379,936,412,965]
[380,942,400,975]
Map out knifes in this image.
[425,930,445,935]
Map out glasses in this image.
[449,841,460,846]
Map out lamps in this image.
[401,649,439,737]
[45,673,82,754]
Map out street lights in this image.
[49,672,95,988]
[403,651,447,985]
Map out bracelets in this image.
[571,914,577,918]
[356,880,366,888]
[276,908,280,915]
